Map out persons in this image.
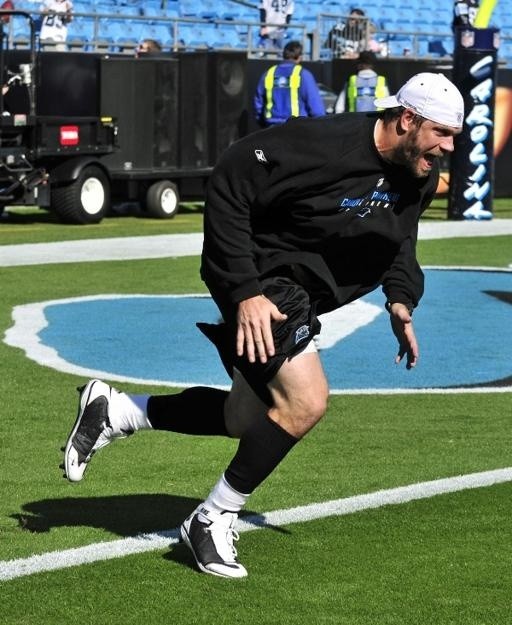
[451,0,481,36]
[363,22,382,52]
[0,0,16,50]
[255,0,296,57]
[132,39,160,57]
[252,40,326,125]
[38,0,74,53]
[335,49,389,116]
[59,71,466,578]
[318,8,369,60]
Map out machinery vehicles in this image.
[0,8,214,222]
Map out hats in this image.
[373,72,465,131]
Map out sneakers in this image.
[180,504,249,580]
[59,379,114,481]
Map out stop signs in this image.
[60,125,79,145]
